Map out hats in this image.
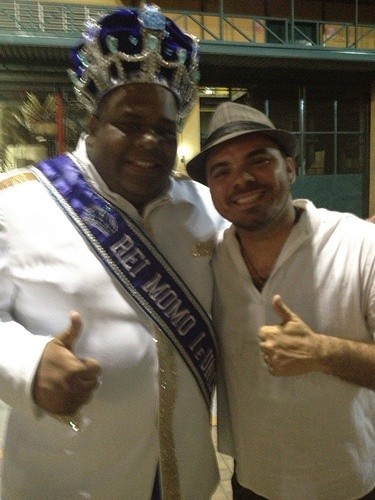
[186,102,299,187]
[68,3,200,128]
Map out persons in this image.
[184,100,374,500]
[2,4,232,496]
